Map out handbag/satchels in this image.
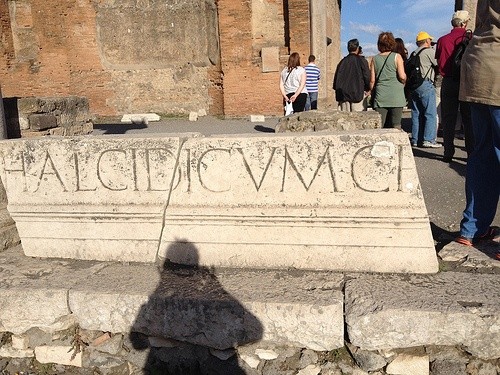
[441,29,472,79]
[371,84,376,101]
[284,100,293,116]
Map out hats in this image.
[416,32,432,43]
[451,10,471,23]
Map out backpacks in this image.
[404,47,432,89]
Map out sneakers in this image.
[422,141,442,147]
[413,143,417,146]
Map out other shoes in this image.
[447,157,452,162]
[455,226,500,245]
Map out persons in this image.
[303,55,321,111]
[333,0,500,259]
[279,52,307,117]
